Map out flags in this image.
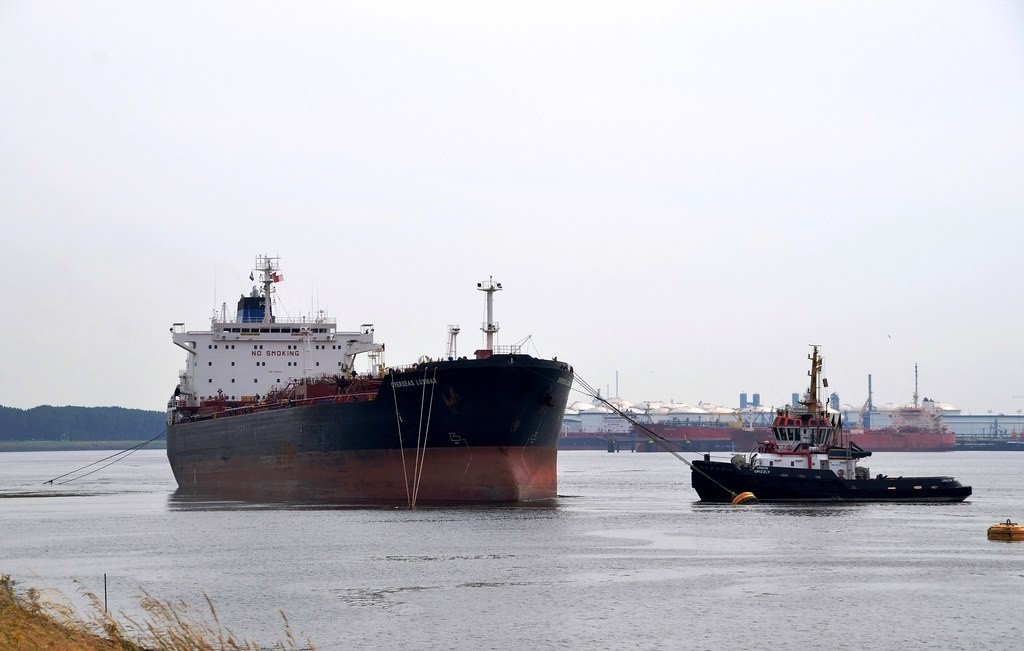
[273,272,280,282]
[249,271,254,281]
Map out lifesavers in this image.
[838,469,845,475]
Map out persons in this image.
[366,329,369,334]
[552,356,557,361]
[777,409,788,416]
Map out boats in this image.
[629,412,739,453]
[692,339,975,505]
[728,363,959,452]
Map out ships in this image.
[162,253,578,513]
